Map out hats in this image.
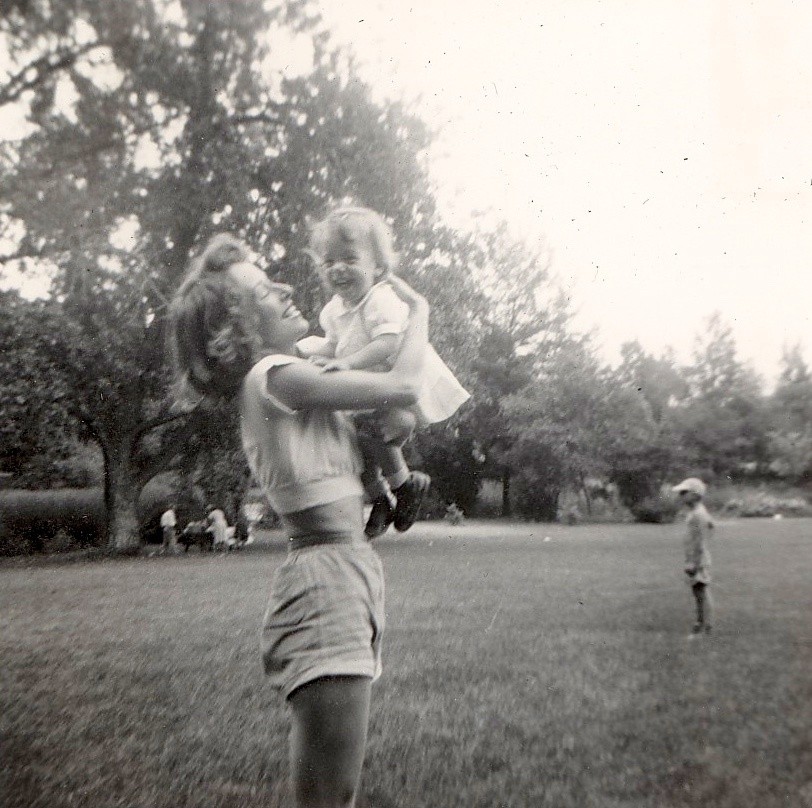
[672,478,705,496]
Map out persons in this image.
[159,502,250,555]
[672,477,716,637]
[167,235,431,808]
[298,207,471,538]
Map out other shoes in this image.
[394,471,431,531]
[688,624,711,639]
[365,494,397,537]
